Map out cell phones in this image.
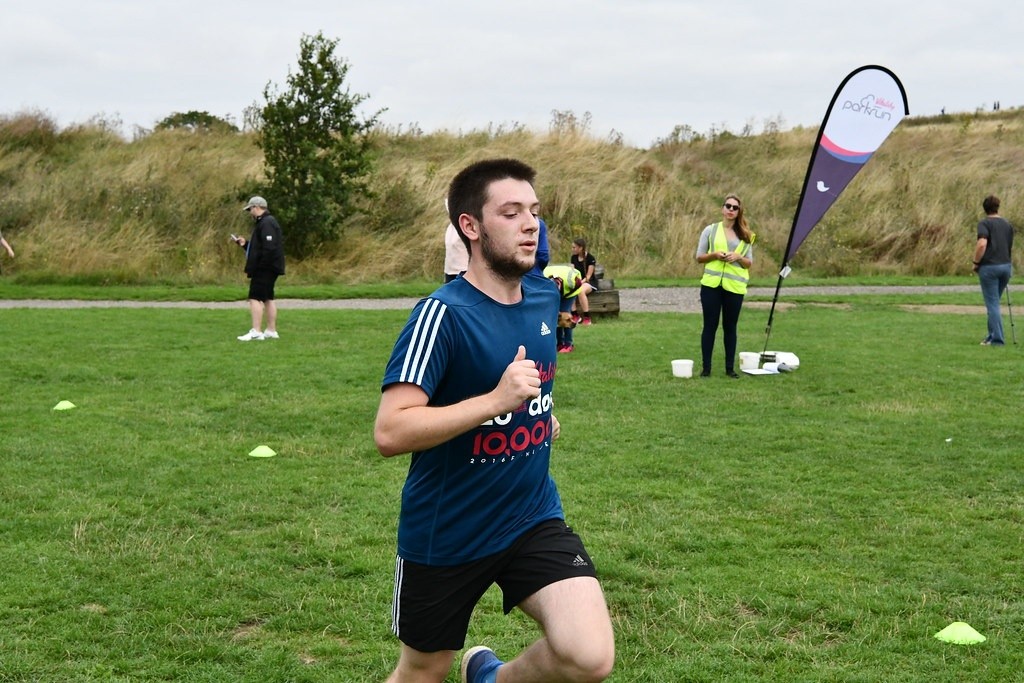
[231,234,237,241]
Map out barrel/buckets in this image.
[671,359,694,378]
[739,352,760,371]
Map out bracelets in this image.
[972,260,979,265]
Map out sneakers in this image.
[582,315,591,326]
[572,314,582,324]
[263,328,280,338]
[461,645,504,683]
[237,328,264,341]
[557,344,574,354]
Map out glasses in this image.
[723,203,739,211]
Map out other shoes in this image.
[726,371,739,378]
[980,339,1002,347]
[700,369,711,377]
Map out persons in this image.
[543,265,582,353]
[570,238,598,326]
[445,198,550,283]
[230,196,286,341]
[972,196,1014,345]
[0,231,14,256]
[375,160,616,683]
[695,193,753,378]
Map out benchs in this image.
[559,265,620,321]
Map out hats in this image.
[243,196,267,210]
